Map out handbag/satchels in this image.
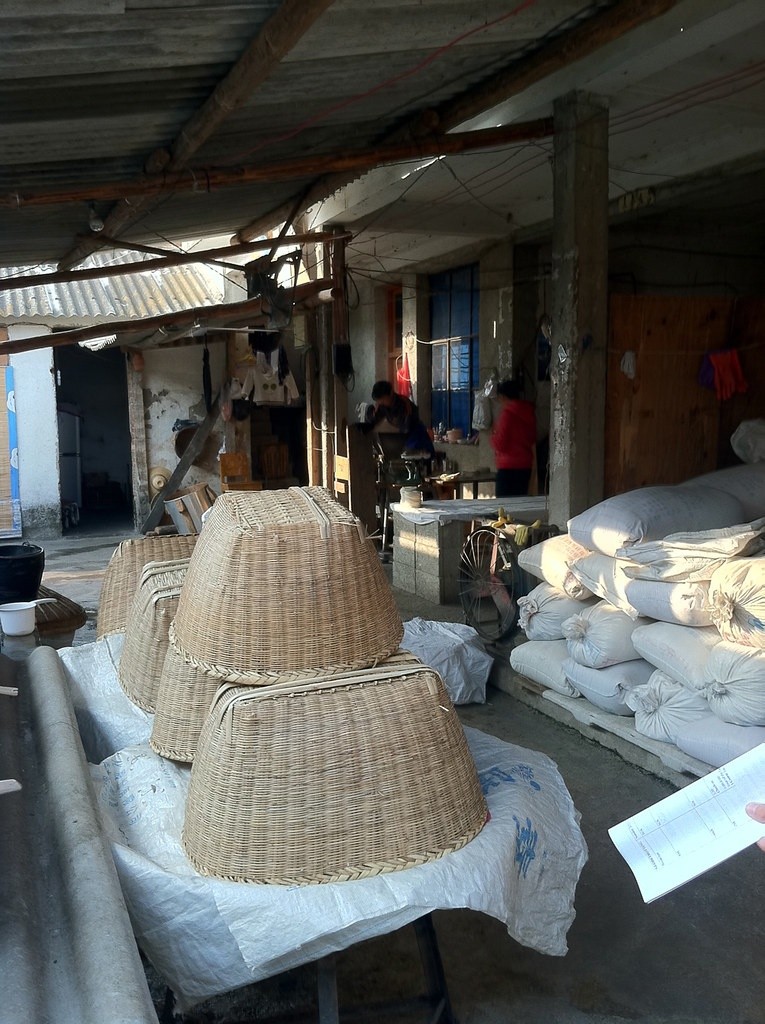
[397,358,412,399]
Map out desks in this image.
[433,471,495,501]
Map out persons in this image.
[365,380,433,455]
[489,380,537,498]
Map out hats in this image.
[148,467,172,502]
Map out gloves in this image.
[491,507,514,528]
[514,519,542,546]
